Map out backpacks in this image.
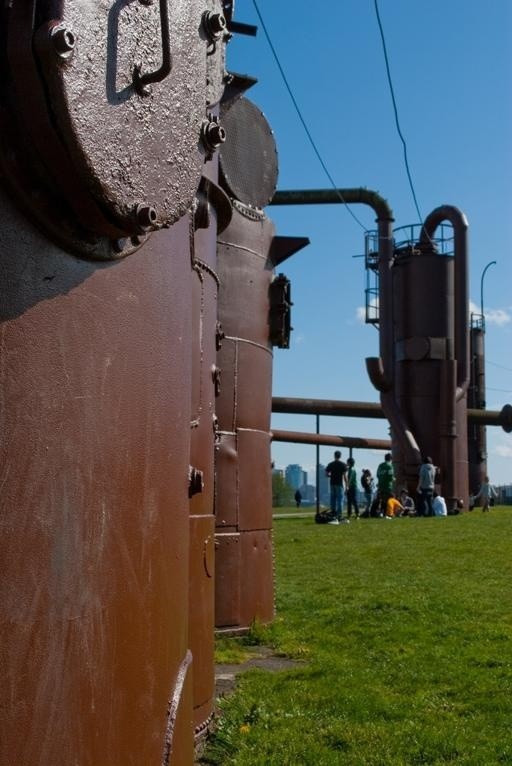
[315,508,337,524]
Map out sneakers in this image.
[327,511,360,524]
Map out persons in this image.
[384,492,406,519]
[430,492,447,517]
[323,451,350,523]
[376,454,396,518]
[345,458,360,521]
[399,488,418,518]
[360,469,374,519]
[370,495,386,518]
[471,475,498,513]
[415,456,437,517]
[294,490,302,508]
